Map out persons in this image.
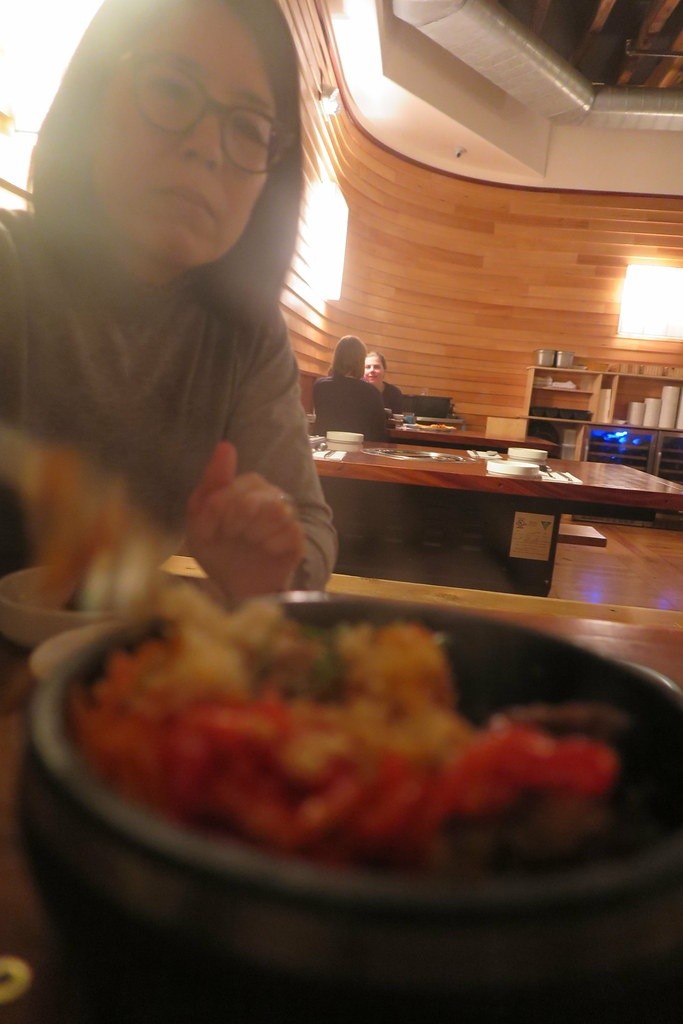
[313,336,387,441]
[359,350,403,414]
[1,0,340,607]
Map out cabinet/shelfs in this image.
[523,365,683,532]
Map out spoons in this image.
[539,464,555,479]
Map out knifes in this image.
[556,472,572,481]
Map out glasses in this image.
[117,45,296,173]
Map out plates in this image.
[420,425,457,432]
[627,386,683,430]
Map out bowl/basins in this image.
[0,565,174,650]
[21,590,683,1024]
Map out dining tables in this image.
[0,411,683,1024]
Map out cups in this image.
[393,414,404,430]
